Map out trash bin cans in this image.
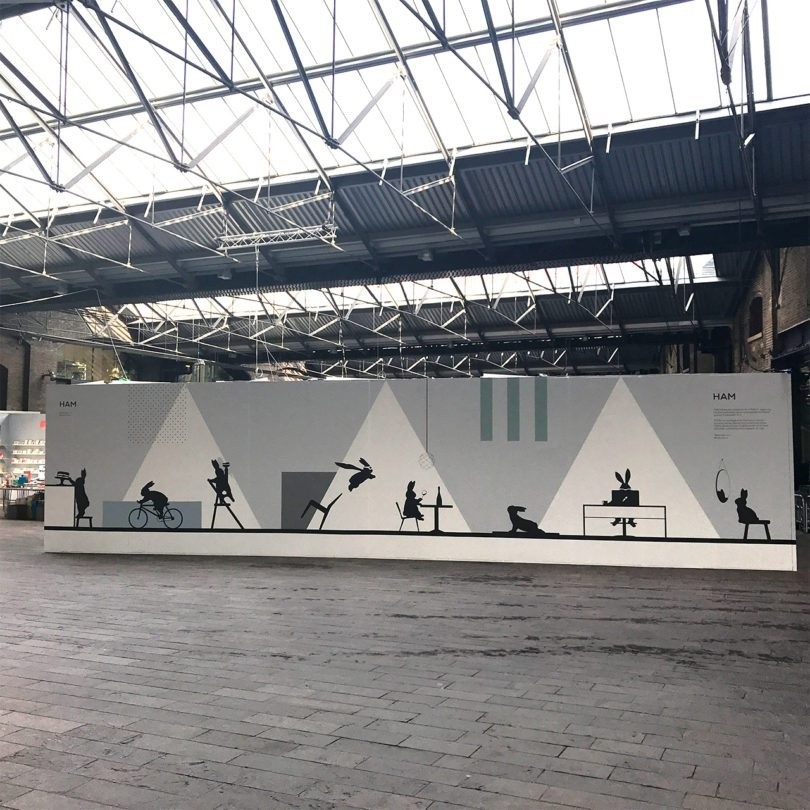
[7,504,26,520]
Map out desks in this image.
[0,487,44,510]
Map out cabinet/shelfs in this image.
[11,444,45,475]
[0,458,8,475]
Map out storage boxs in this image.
[6,504,26,519]
